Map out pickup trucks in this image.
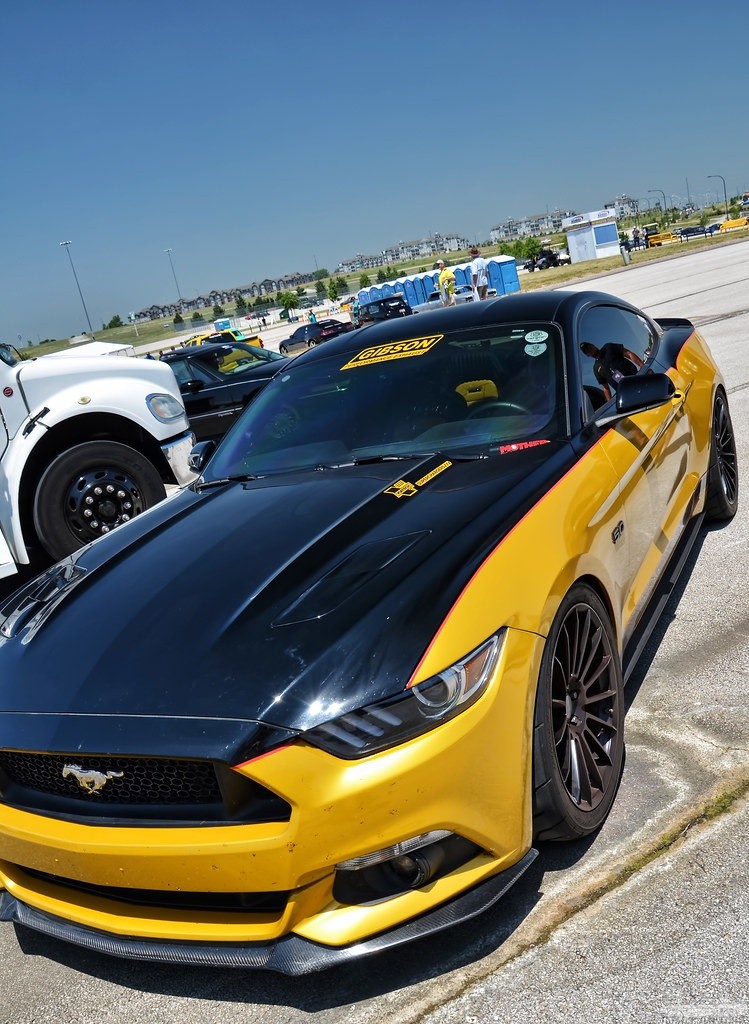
[183,330,264,373]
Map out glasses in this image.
[437,263,441,266]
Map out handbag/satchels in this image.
[443,283,454,305]
[472,286,480,302]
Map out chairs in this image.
[470,352,548,418]
[354,343,467,448]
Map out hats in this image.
[580,342,591,355]
[435,259,444,264]
[468,248,481,257]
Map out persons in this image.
[180,341,185,348]
[433,259,456,307]
[159,351,163,361]
[351,297,361,318]
[257,317,262,331]
[144,351,155,360]
[262,316,267,325]
[170,346,175,351]
[470,248,489,300]
[308,308,317,323]
[579,342,645,402]
[632,225,641,251]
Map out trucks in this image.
[1,337,203,581]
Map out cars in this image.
[159,341,306,451]
[279,319,356,355]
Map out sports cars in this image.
[1,290,739,980]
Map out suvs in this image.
[357,296,412,328]
[411,285,475,315]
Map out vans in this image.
[680,225,707,239]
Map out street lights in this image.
[707,175,729,221]
[163,249,185,312]
[648,190,669,221]
[59,241,95,341]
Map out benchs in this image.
[706,216,749,237]
[681,226,707,243]
[648,232,679,248]
[623,237,645,252]
